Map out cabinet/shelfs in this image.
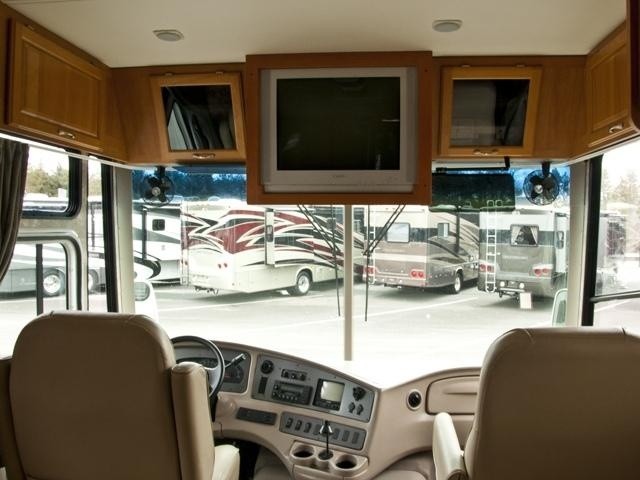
[1,2,127,165]
[570,22,635,158]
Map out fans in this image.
[141,165,174,208]
[522,161,560,206]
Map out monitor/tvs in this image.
[259,68,417,194]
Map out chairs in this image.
[0,312,241,480]
[431,324,640,480]
